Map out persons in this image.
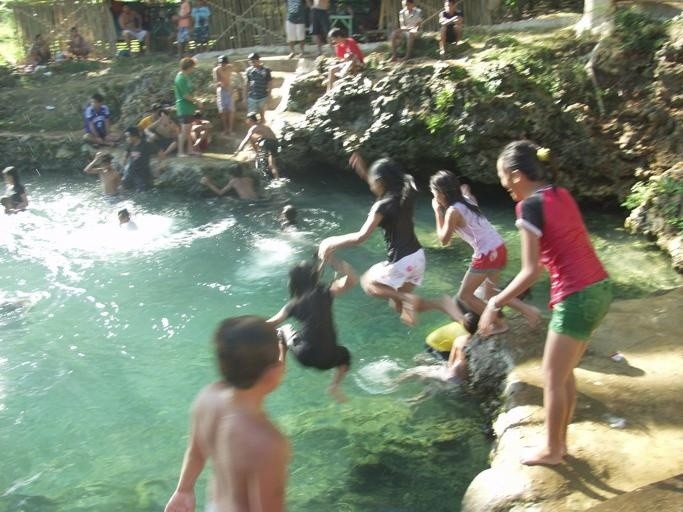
[322,28,363,90]
[285,0,331,59]
[389,1,424,60]
[175,57,202,157]
[117,4,151,55]
[1,166,28,215]
[162,314,295,512]
[173,1,211,59]
[66,27,91,58]
[282,205,305,228]
[209,55,241,137]
[446,309,480,383]
[241,52,272,123]
[477,137,613,467]
[83,92,213,194]
[200,111,286,201]
[429,168,543,336]
[316,150,468,331]
[438,1,464,58]
[118,209,137,229]
[262,251,359,405]
[29,33,51,65]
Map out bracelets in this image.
[487,296,501,313]
